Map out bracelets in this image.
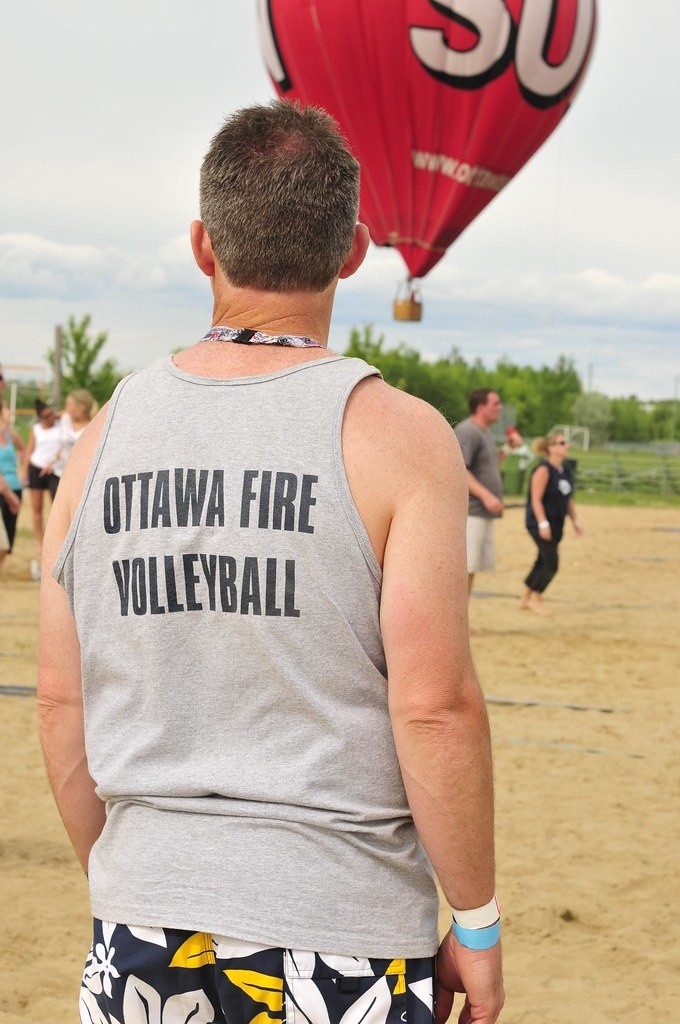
[539,521,549,528]
[449,897,501,930]
[450,919,501,950]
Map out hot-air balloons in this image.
[254,1,599,321]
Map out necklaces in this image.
[200,324,327,352]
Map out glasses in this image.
[553,441,566,447]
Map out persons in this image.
[21,399,60,579]
[454,388,583,615]
[0,376,26,565]
[37,98,506,1024]
[39,390,100,499]
